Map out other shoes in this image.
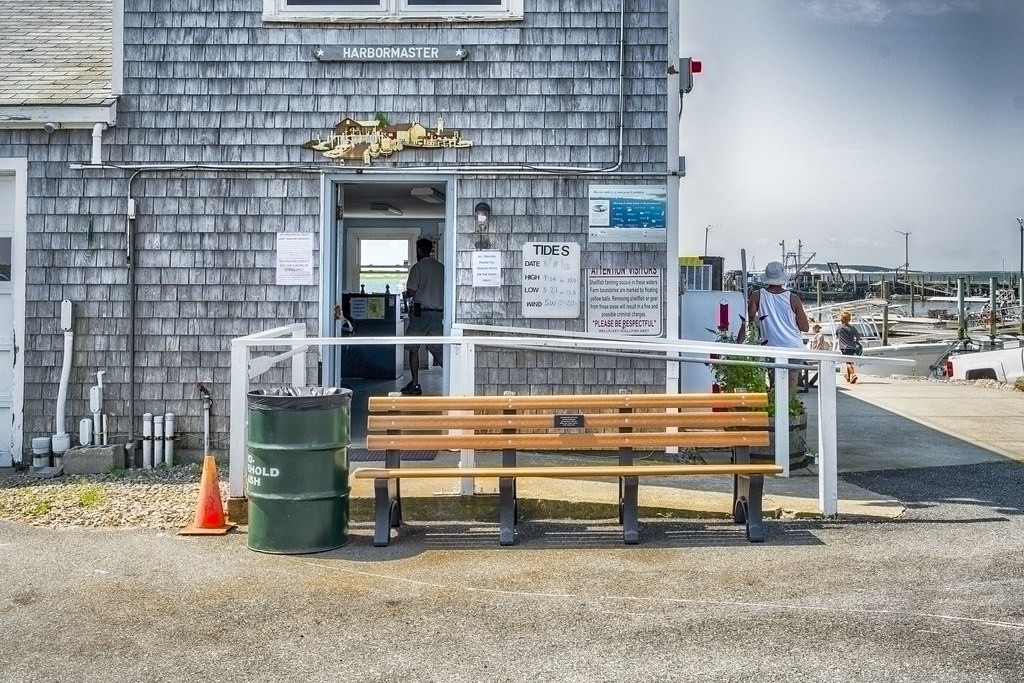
[844,374,850,382]
[850,375,858,384]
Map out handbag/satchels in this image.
[854,341,863,356]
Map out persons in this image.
[963,284,1013,338]
[790,276,957,303]
[806,324,825,387]
[834,311,860,383]
[398,238,444,394]
[749,262,809,399]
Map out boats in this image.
[800,296,1024,381]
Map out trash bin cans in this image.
[247,386,354,554]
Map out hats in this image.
[759,261,792,287]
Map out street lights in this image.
[894,230,911,292]
[705,224,721,255]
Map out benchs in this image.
[355,393,782,548]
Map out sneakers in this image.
[401,381,422,394]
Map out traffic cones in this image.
[178,455,236,535]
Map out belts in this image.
[420,308,443,312]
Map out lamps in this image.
[474,203,491,235]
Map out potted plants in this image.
[702,313,808,471]
